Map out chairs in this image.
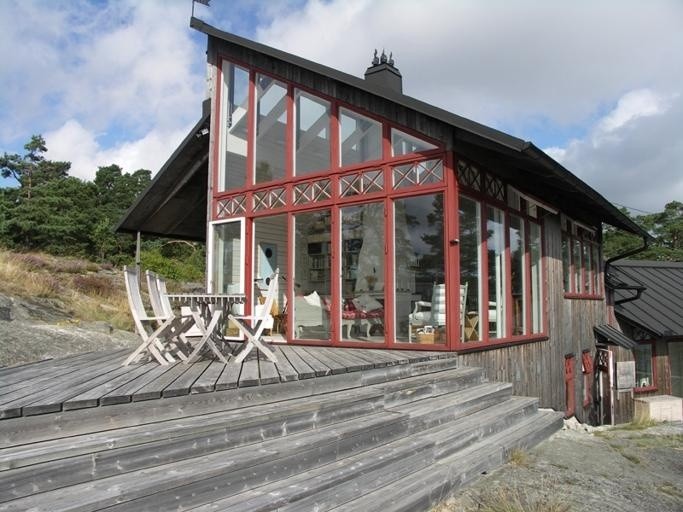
[123,265,280,366]
[322,292,385,339]
[406,280,468,345]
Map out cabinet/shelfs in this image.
[308,231,362,297]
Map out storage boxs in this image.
[415,332,435,345]
[633,394,683,422]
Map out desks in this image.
[512,293,523,335]
[465,311,479,340]
